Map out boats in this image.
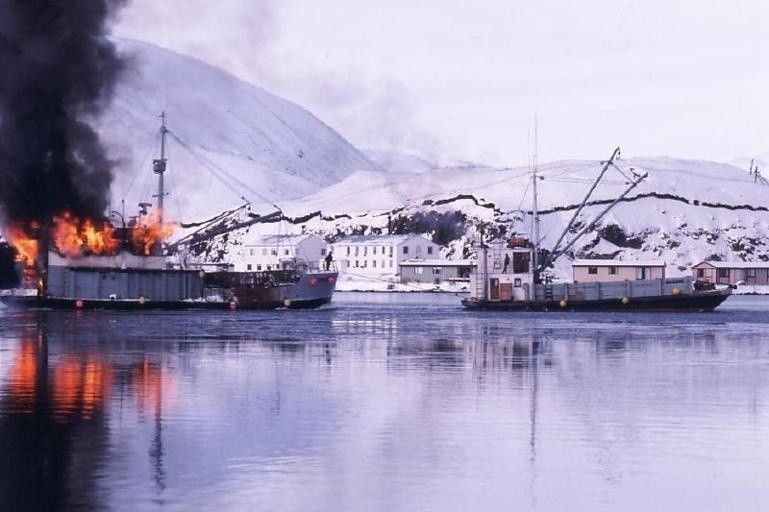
[460,117,734,313]
[0,110,338,309]
